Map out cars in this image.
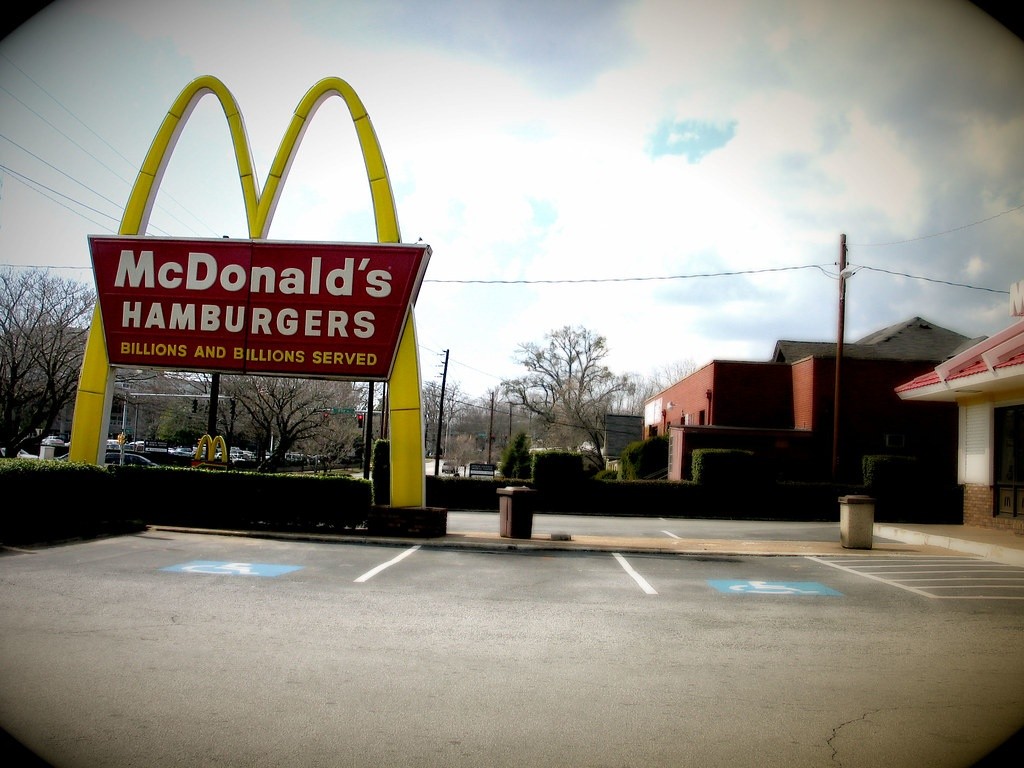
[168,446,271,464]
[107,439,145,453]
[41,435,71,447]
[442,460,458,474]
[105,453,157,466]
[1,447,39,459]
[285,451,303,462]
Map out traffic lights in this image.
[120,434,128,445]
[358,414,364,429]
[192,399,198,413]
[229,400,236,419]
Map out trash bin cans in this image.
[496,486,538,538]
[838,494,877,549]
